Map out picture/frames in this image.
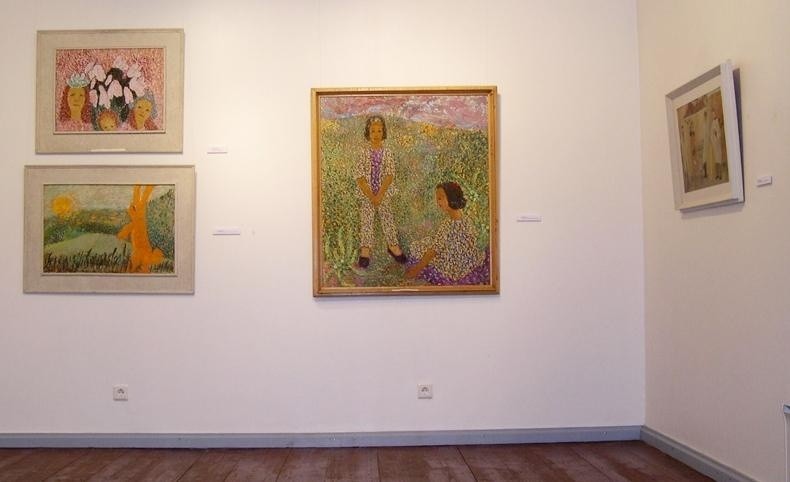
[665,59,744,211]
[22,163,197,296]
[310,83,501,296]
[35,29,183,153]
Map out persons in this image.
[60,71,94,128]
[404,182,483,281]
[99,108,121,130]
[351,114,409,270]
[122,98,159,130]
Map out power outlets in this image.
[113,386,128,401]
[417,385,431,399]
